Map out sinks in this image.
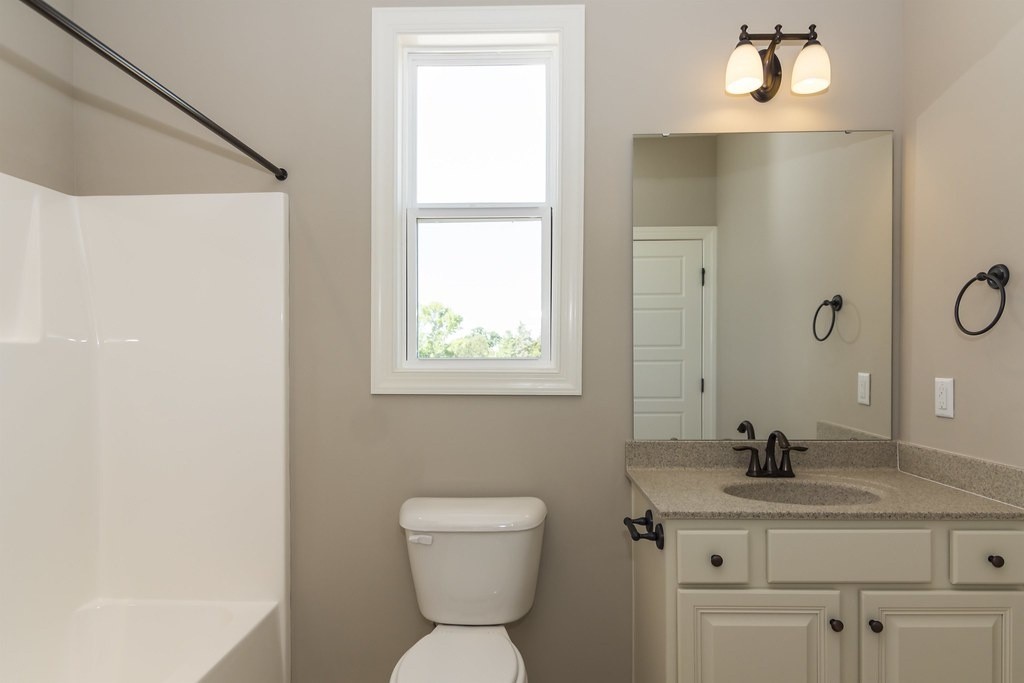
[720,478,883,507]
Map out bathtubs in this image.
[0,594,286,682]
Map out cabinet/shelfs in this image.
[630,483,1024,683]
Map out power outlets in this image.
[857,372,870,406]
[934,377,954,419]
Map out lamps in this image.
[724,23,832,102]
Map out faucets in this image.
[763,430,791,474]
[736,420,756,440]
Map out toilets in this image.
[387,495,548,683]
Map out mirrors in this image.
[630,128,895,442]
